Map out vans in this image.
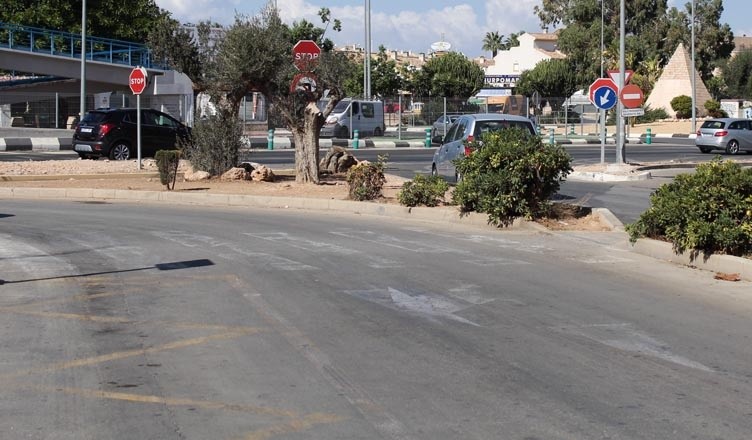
[316,99,385,139]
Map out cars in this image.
[695,117,752,155]
[431,113,538,186]
[430,114,461,143]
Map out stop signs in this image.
[621,85,644,109]
[290,40,321,72]
[128,69,145,95]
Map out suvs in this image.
[71,107,197,161]
